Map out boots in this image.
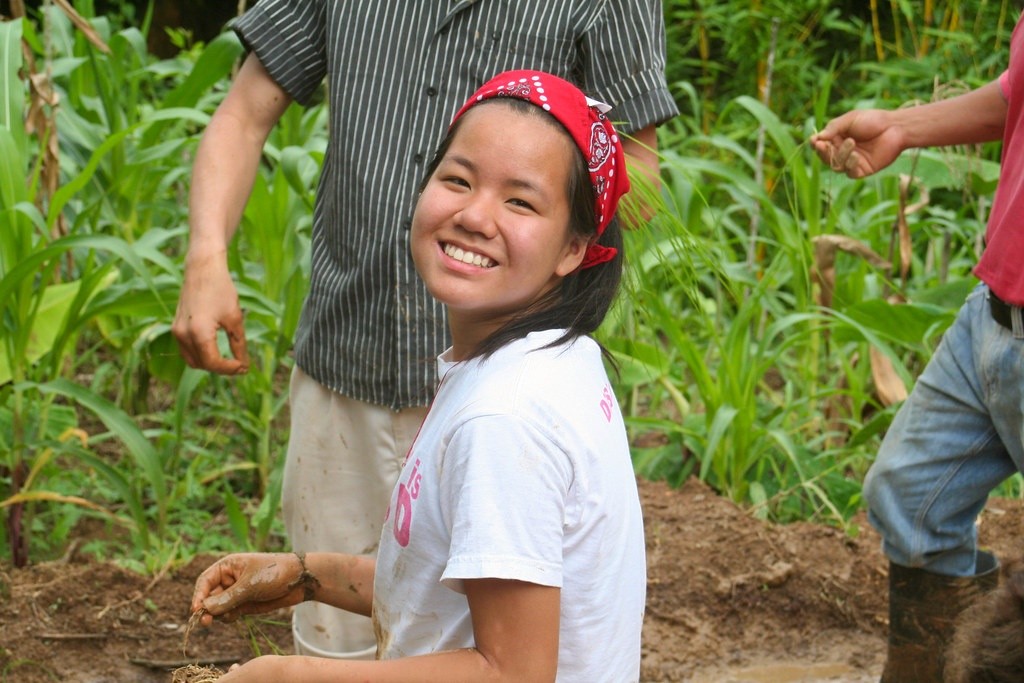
[881,550,1001,682]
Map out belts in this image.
[986,288,1024,330]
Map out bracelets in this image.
[287,551,321,601]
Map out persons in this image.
[171,0,683,660]
[810,7,1024,683]
[189,69,648,683]
